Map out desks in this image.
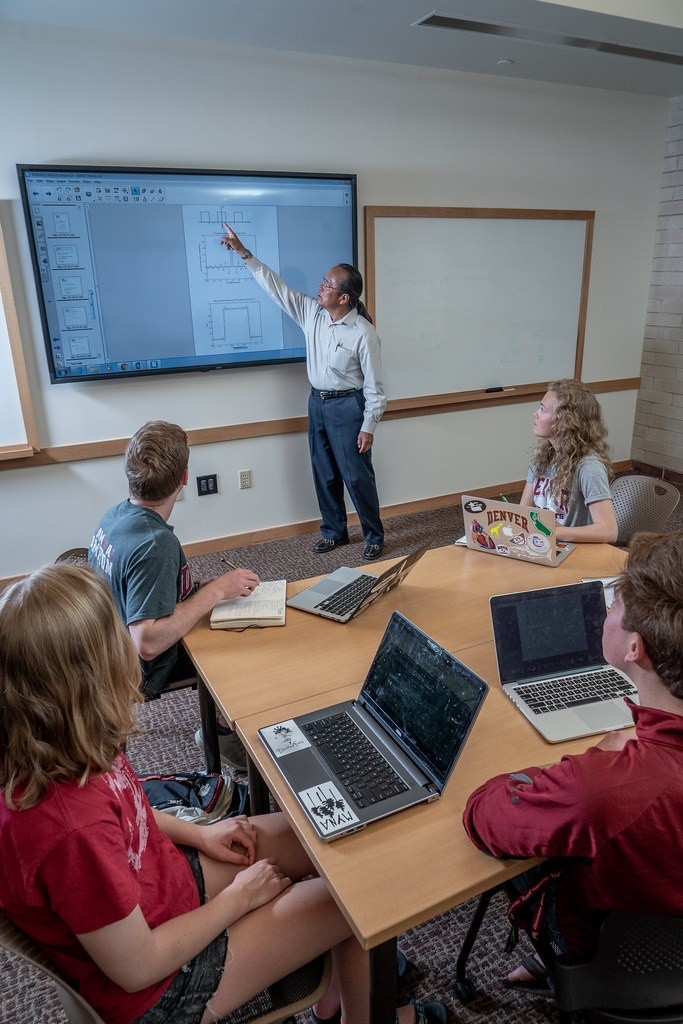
[180,546,644,1024]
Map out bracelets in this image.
[241,249,251,260]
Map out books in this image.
[210,579,286,630]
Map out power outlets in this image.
[196,474,218,495]
[238,470,251,489]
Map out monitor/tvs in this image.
[17,164,358,384]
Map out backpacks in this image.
[136,772,250,826]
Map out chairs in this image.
[458,885,682,1024]
[587,476,680,547]
[51,547,199,700]
[0,935,333,1024]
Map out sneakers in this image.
[195,726,249,770]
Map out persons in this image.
[88,420,260,683]
[220,222,387,560]
[0,563,448,1024]
[462,528,683,998]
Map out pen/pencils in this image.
[220,558,261,587]
[500,493,508,502]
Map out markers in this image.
[486,387,515,392]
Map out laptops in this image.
[461,494,577,567]
[258,611,489,843]
[287,540,434,623]
[489,581,639,744]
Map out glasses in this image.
[321,277,345,293]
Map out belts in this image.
[310,388,358,400]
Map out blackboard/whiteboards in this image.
[0,227,40,460]
[364,206,596,413]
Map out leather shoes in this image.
[363,542,384,558]
[316,539,350,552]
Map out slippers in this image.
[394,999,448,1024]
[501,955,555,993]
[310,948,406,1023]
